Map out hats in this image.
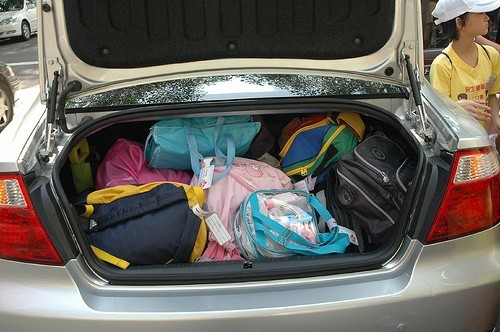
[431,0,500,26]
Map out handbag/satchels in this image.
[97,137,194,194]
[140,115,263,182]
[189,153,295,261]
[74,180,210,269]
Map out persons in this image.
[430,0,500,136]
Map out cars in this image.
[0,0,500,332]
[0,0,38,41]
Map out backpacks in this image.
[232,110,415,261]
